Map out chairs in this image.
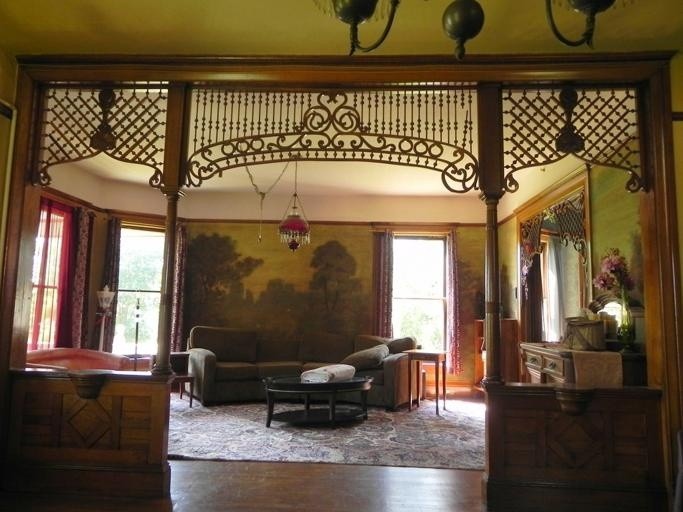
[167,351,195,408]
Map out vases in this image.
[619,331,636,352]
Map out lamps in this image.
[274,145,312,252]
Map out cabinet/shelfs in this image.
[517,339,576,386]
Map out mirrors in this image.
[512,163,592,343]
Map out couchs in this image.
[187,324,424,415]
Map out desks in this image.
[400,348,450,416]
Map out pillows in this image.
[340,333,418,368]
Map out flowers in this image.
[594,245,642,332]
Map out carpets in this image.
[165,391,487,471]
